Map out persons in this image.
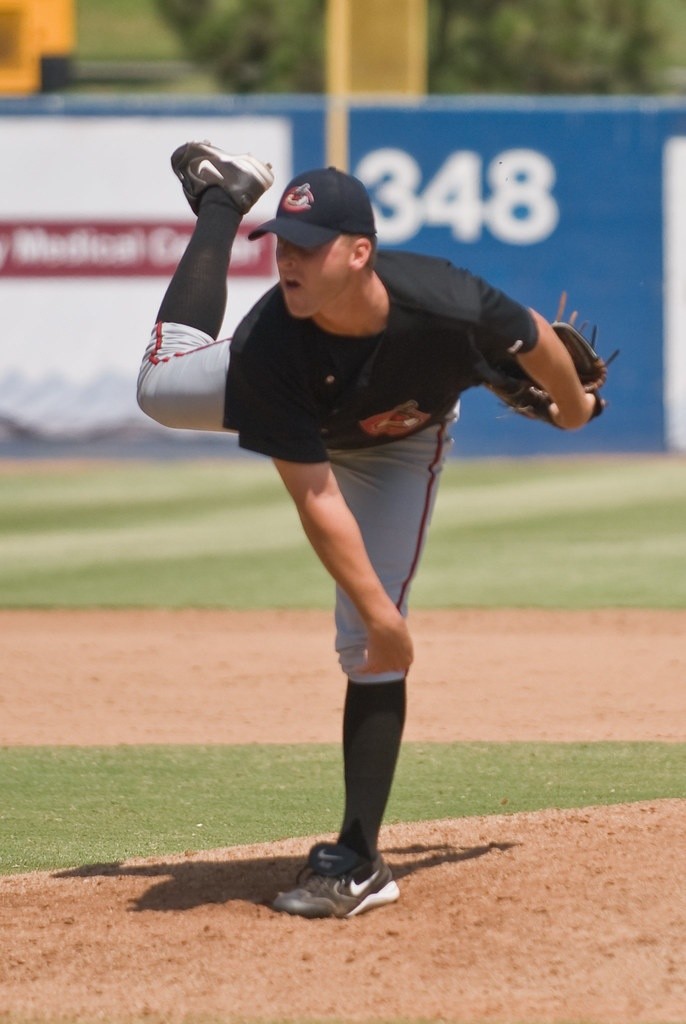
[136,141,607,917]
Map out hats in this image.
[248,167,375,248]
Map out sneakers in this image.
[273,843,400,919]
[171,143,274,217]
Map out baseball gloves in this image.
[482,320,610,431]
